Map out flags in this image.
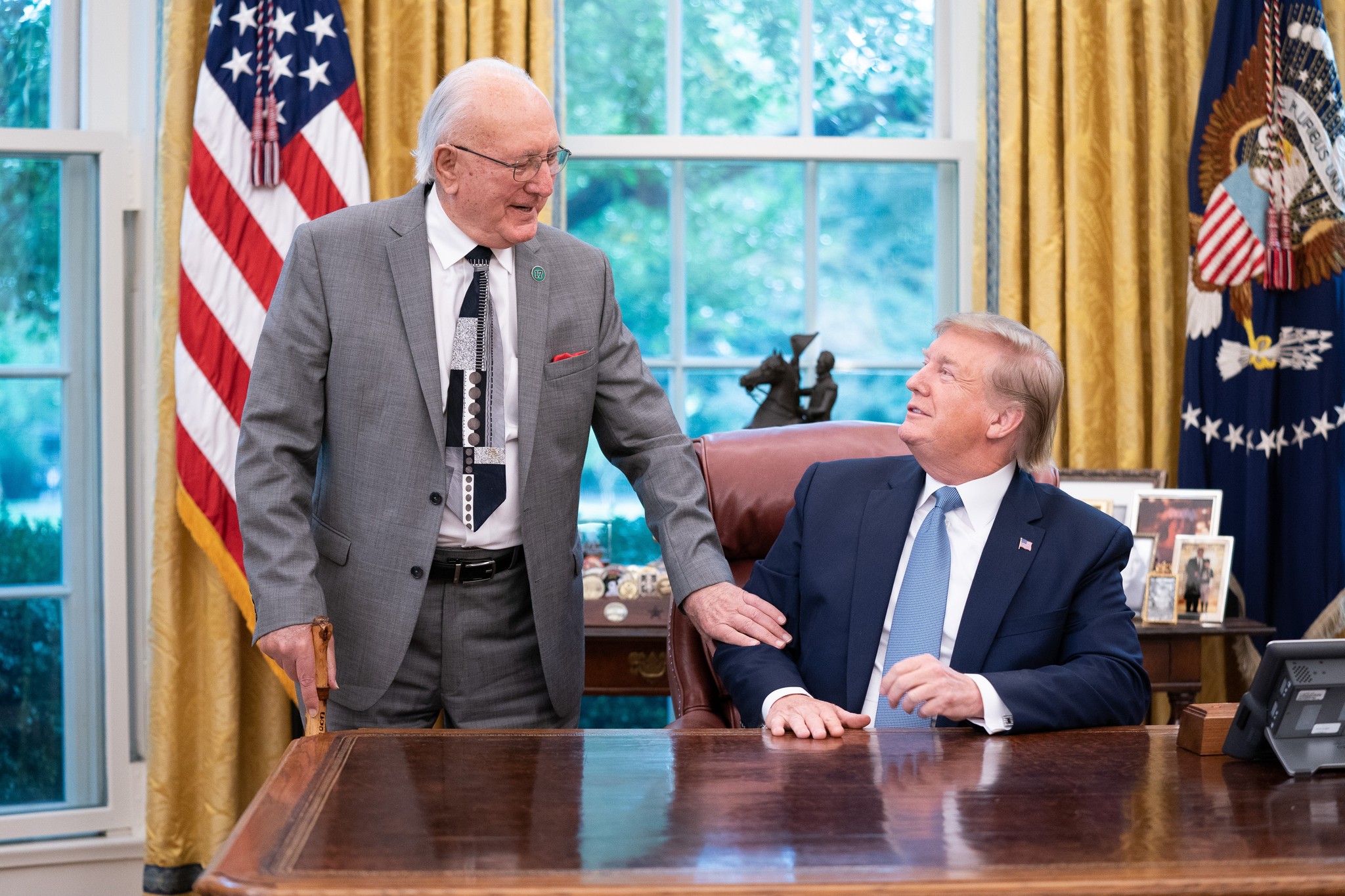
[178,0,370,709]
[1175,0,1345,686]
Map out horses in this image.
[741,348,805,429]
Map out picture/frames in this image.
[1063,475,1234,624]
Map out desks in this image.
[194,725,1345,895]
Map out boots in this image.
[1200,601,1203,613]
[1205,602,1208,612]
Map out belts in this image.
[427,549,526,586]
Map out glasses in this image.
[447,144,572,182]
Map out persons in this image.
[711,312,1152,740]
[233,55,795,735]
[1184,548,1214,612]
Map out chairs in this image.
[667,420,1058,729]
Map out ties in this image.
[1198,558,1201,573]
[873,485,964,729]
[445,245,507,534]
[878,731,947,872]
